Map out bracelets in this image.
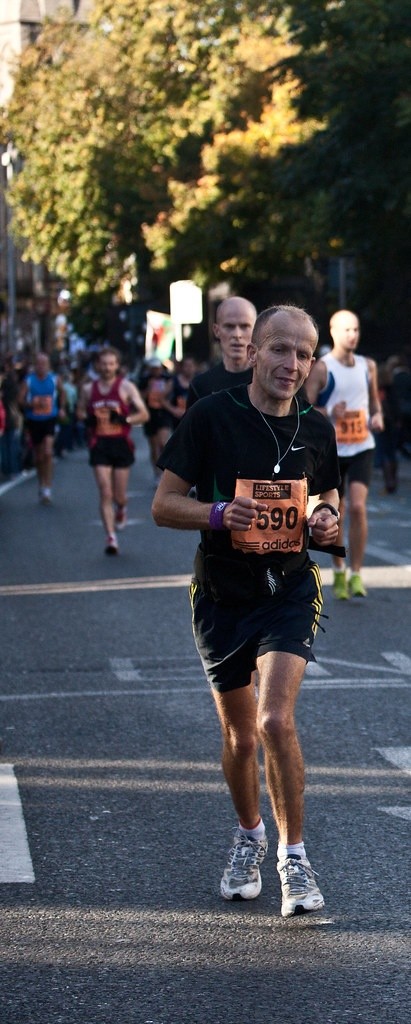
[209,502,228,529]
[313,503,340,521]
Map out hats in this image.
[149,359,161,368]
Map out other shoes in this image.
[104,536,119,556]
[39,486,53,507]
[380,488,395,495]
[115,507,128,531]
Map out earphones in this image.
[271,472,279,482]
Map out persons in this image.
[17,353,65,503]
[78,348,150,554]
[139,358,210,498]
[185,295,258,411]
[305,310,384,600]
[378,356,411,495]
[152,305,340,916]
[0,350,136,485]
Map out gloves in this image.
[110,410,129,425]
[84,415,98,428]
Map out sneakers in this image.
[221,826,268,901]
[333,571,349,601]
[348,575,367,598]
[276,854,325,918]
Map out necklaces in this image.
[251,396,300,473]
